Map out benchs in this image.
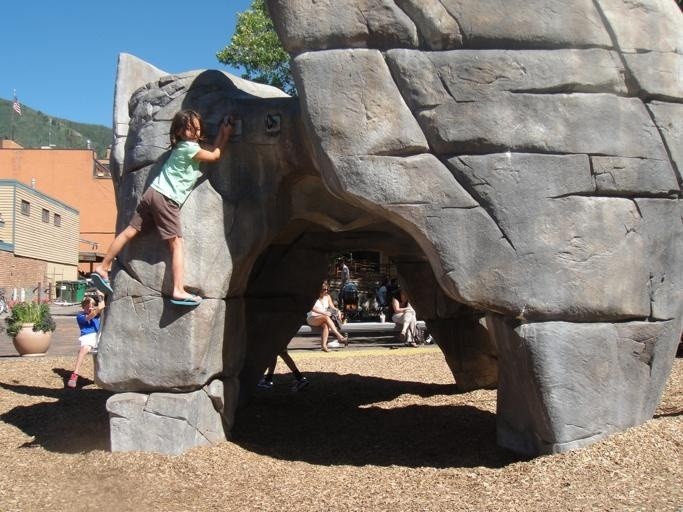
[298,321,427,346]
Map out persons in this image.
[305,280,348,354]
[87,107,235,308]
[255,348,310,392]
[388,289,419,348]
[64,291,106,391]
[327,256,400,323]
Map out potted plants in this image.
[4,299,56,357]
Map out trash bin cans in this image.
[56,281,87,303]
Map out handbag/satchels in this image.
[329,313,342,330]
[415,323,426,344]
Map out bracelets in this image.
[214,144,225,153]
[92,310,97,316]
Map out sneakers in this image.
[68,373,78,388]
[399,333,418,348]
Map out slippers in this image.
[91,272,114,295]
[170,294,202,307]
